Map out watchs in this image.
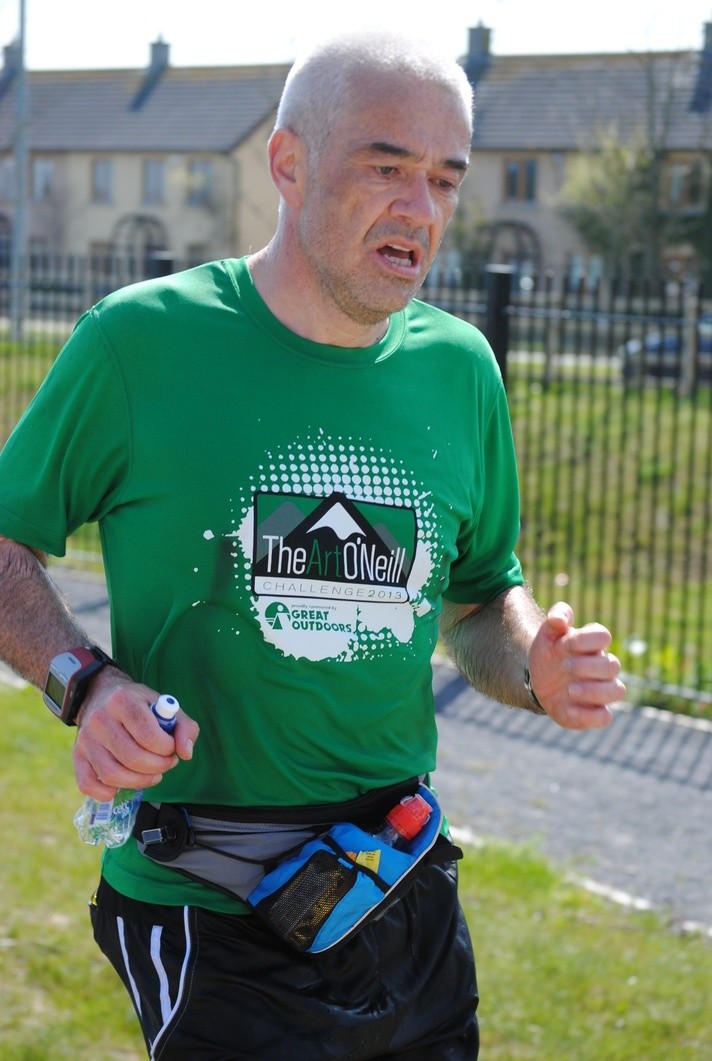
[42,646,122,727]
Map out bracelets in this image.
[523,662,547,716]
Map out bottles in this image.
[72,694,181,849]
[269,793,434,954]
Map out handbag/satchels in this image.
[131,772,467,961]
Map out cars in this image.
[608,316,712,389]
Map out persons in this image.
[1,21,629,1061]
